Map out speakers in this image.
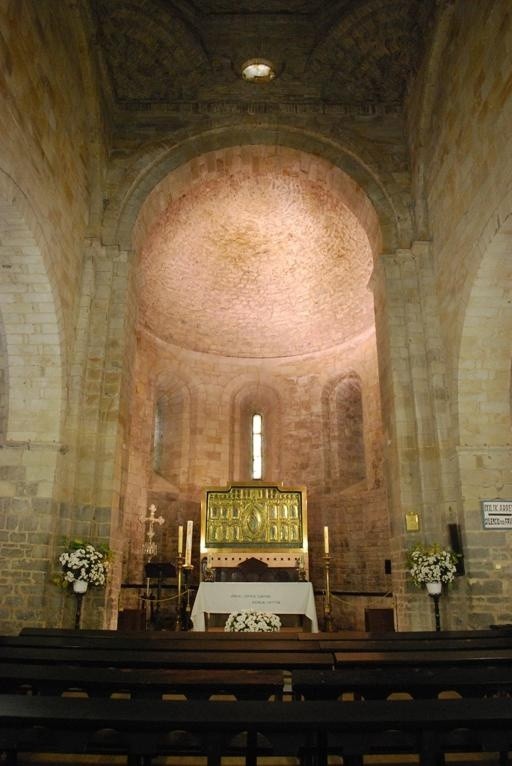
[448,524,464,576]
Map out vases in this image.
[426,580,445,598]
[72,578,91,596]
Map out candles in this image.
[177,524,184,556]
[323,525,329,555]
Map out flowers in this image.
[53,537,112,586]
[407,542,461,583]
[221,606,284,635]
